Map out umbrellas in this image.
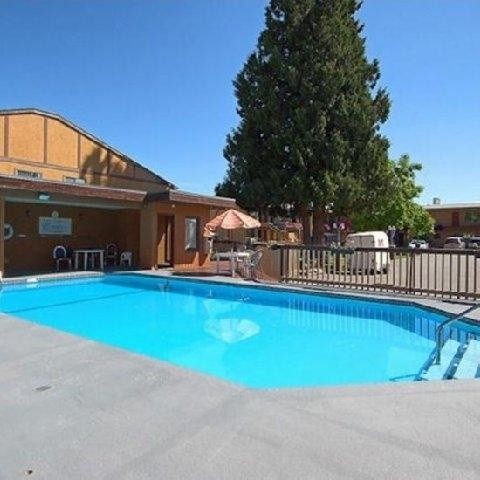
[206,208,262,277]
[202,317,260,344]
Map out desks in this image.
[73,249,105,271]
[214,251,249,277]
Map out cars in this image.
[408,237,480,248]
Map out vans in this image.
[346,231,390,272]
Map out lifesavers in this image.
[4,224,13,239]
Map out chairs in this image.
[235,244,265,279]
[53,244,72,271]
[104,243,132,268]
